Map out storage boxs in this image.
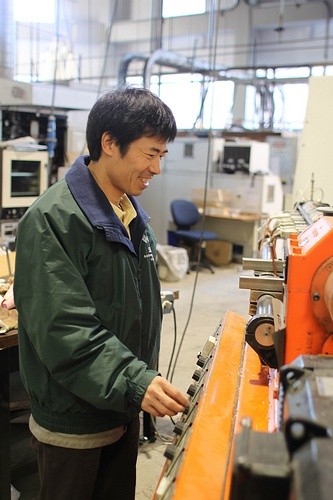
[193,188,233,208]
[195,241,233,264]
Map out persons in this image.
[13,88,190,500]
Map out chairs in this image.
[170,199,219,275]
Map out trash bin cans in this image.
[158,244,189,282]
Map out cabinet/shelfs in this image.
[2,150,49,209]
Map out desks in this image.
[191,209,270,264]
[0,290,180,500]
